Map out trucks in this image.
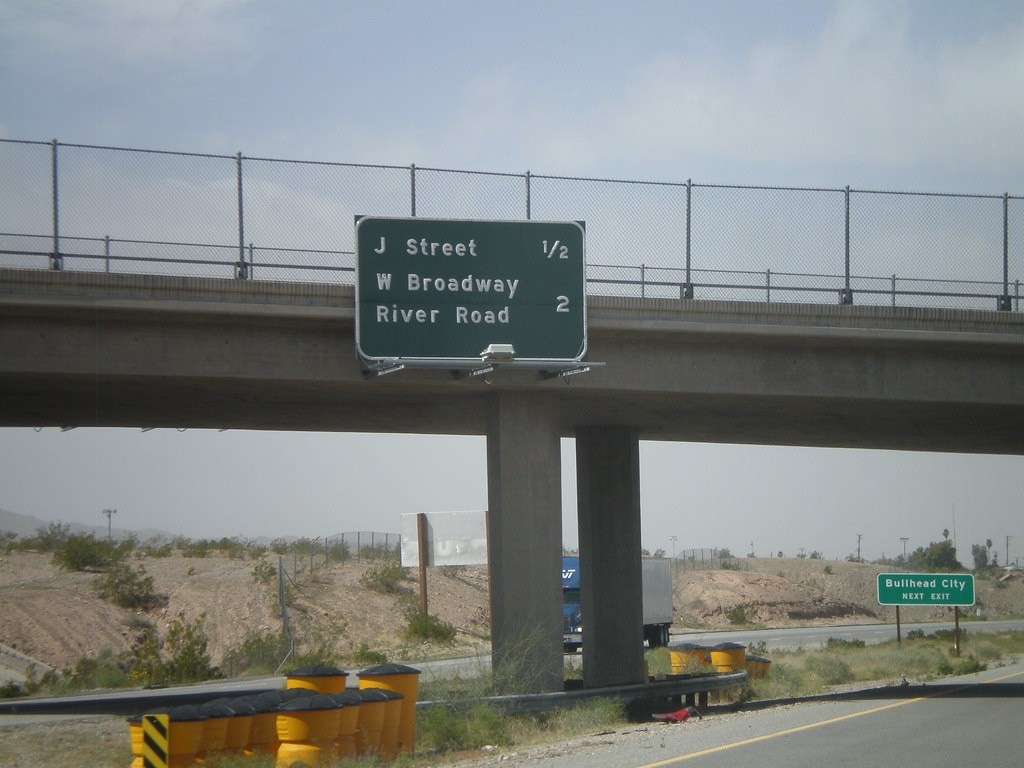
[559,556,673,655]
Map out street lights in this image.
[101,506,117,543]
